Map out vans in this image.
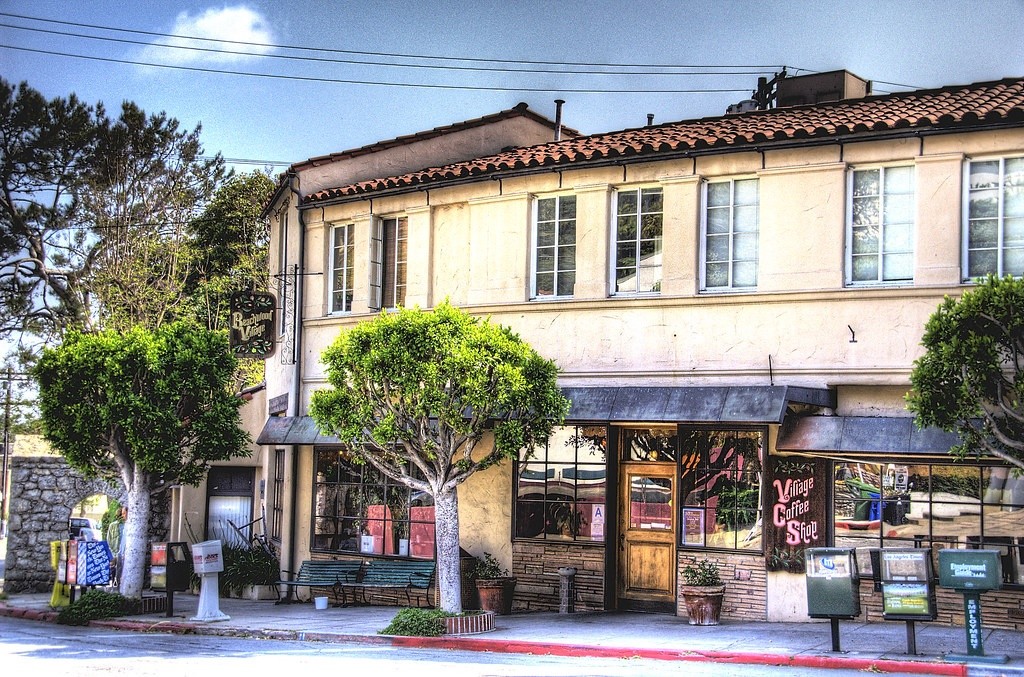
[67,517,91,540]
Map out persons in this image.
[106,506,128,585]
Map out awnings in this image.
[422,386,788,426]
[256,412,455,450]
[773,412,1021,460]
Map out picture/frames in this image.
[682,508,706,547]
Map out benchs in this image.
[273,561,363,605]
[342,561,436,609]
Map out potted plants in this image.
[389,485,412,555]
[351,489,382,553]
[680,559,726,624]
[465,551,517,614]
